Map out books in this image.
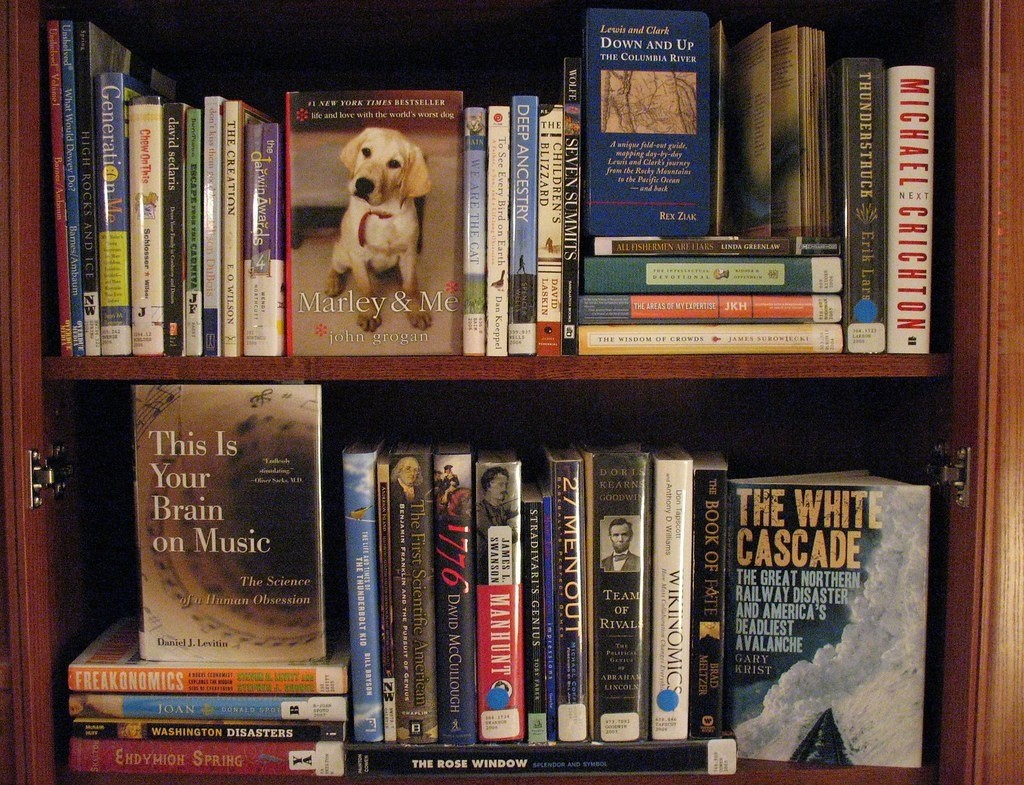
[48,8,936,776]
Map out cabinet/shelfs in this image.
[0,0,1024,785]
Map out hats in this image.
[437,471,442,474]
[443,465,453,470]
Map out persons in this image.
[601,519,638,571]
[477,466,518,583]
[391,456,426,502]
[433,470,443,496]
[438,464,458,499]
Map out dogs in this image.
[322,125,436,335]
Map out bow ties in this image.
[614,554,627,563]
